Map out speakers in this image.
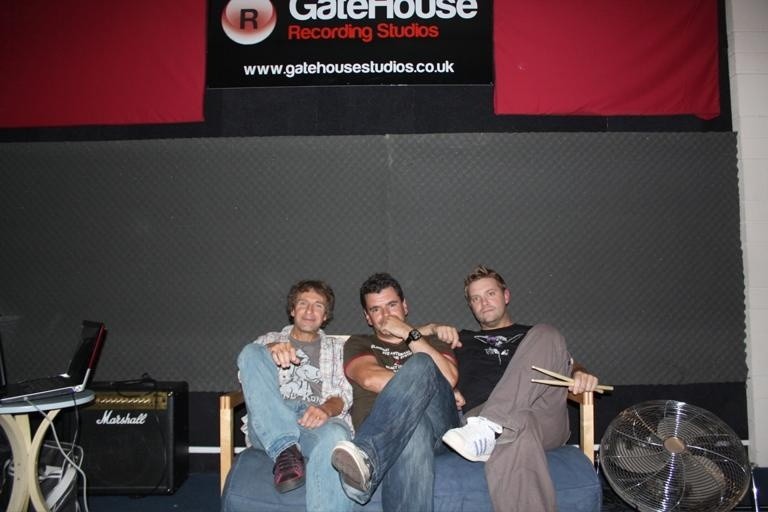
[71,379,190,493]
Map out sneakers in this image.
[442,416,503,462]
[272,444,305,494]
[330,440,376,505]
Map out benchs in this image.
[215,334,599,511]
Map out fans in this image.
[595,397,751,508]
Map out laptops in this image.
[0,321,106,404]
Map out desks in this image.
[1,391,97,511]
